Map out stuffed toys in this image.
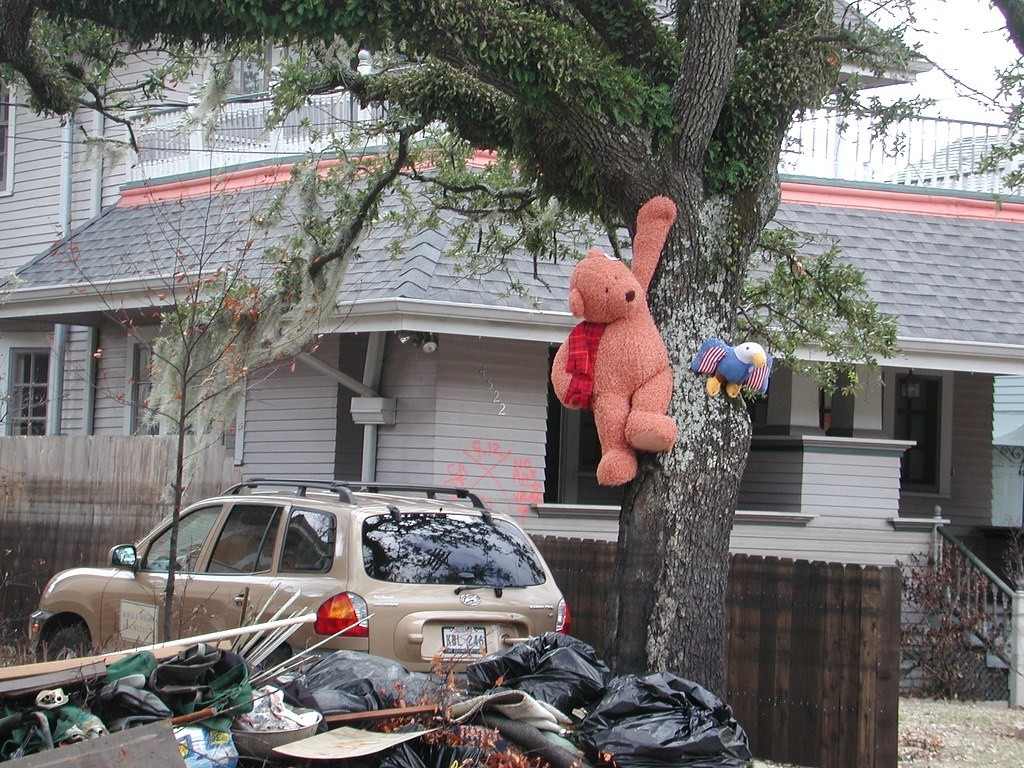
[691,337,773,399]
[550,195,678,488]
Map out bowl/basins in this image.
[231,707,323,757]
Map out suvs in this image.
[27,474,572,683]
